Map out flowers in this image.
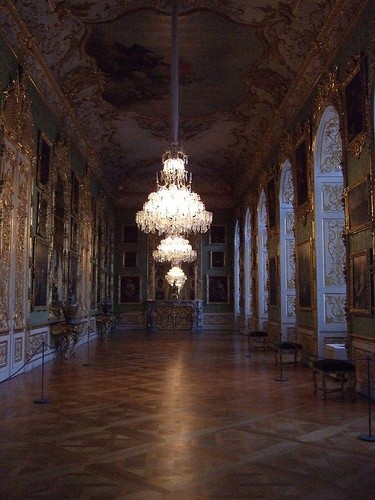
[49,273,58,291]
[101,298,112,306]
[65,295,77,305]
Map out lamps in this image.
[136,0,212,298]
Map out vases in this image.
[102,303,114,315]
[63,305,79,322]
[48,286,62,317]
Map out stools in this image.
[245,332,271,357]
[273,342,303,381]
[313,360,355,398]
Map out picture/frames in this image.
[205,273,230,305]
[118,272,143,304]
[209,249,227,269]
[30,131,80,313]
[123,250,139,268]
[121,223,140,246]
[208,224,230,246]
[240,54,375,319]
